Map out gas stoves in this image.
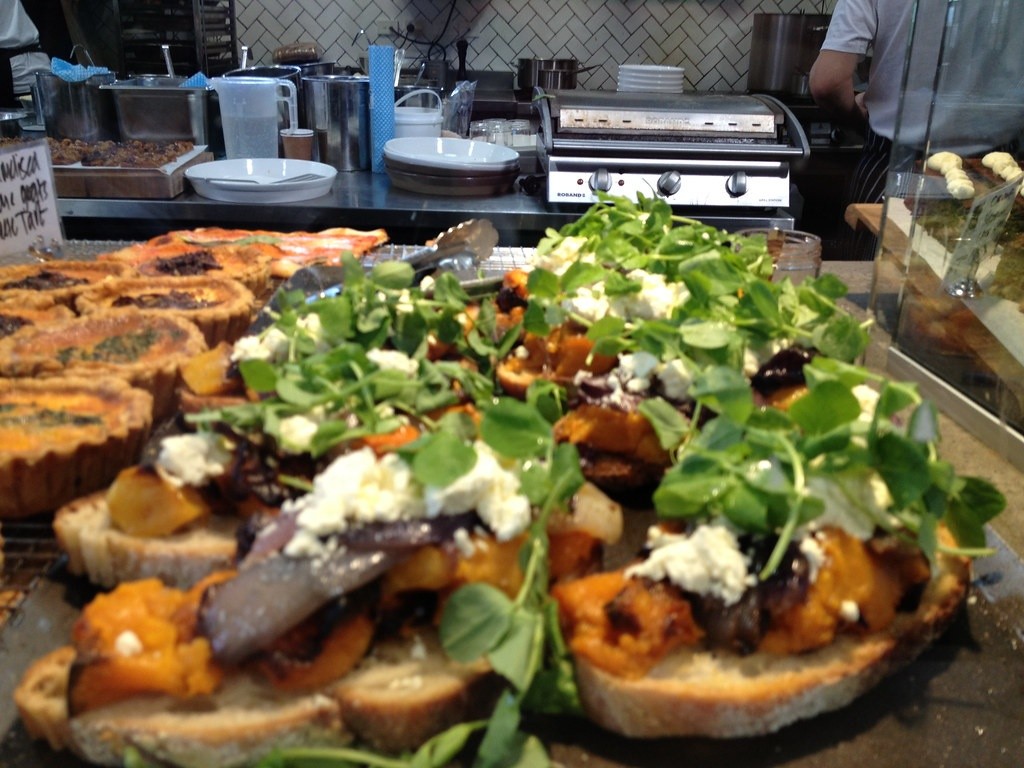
[795,106,865,149]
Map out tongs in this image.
[290,218,498,306]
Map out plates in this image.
[184,156,337,206]
[614,62,685,94]
[383,133,521,197]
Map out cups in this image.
[392,89,443,137]
[738,228,822,285]
[279,128,313,162]
[206,75,299,160]
[301,71,369,173]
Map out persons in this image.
[806,0,1023,265]
[0,0,55,143]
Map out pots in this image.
[30,61,342,145]
[746,12,836,107]
[509,56,602,98]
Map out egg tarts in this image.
[0,246,274,520]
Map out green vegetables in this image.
[204,177,1007,768]
